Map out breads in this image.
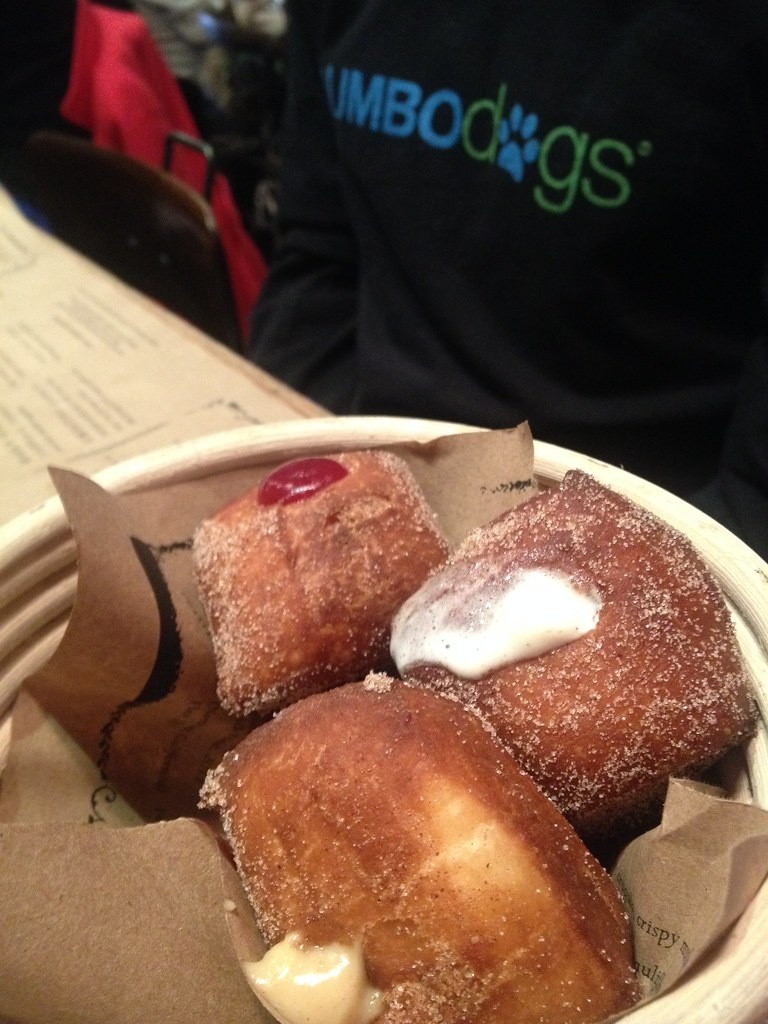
[386,467,761,845]
[200,670,641,1024]
[192,449,454,722]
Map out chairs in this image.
[23,134,240,353]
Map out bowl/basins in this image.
[0,413,768,1024]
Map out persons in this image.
[0,0,768,557]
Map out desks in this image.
[0,183,339,531]
